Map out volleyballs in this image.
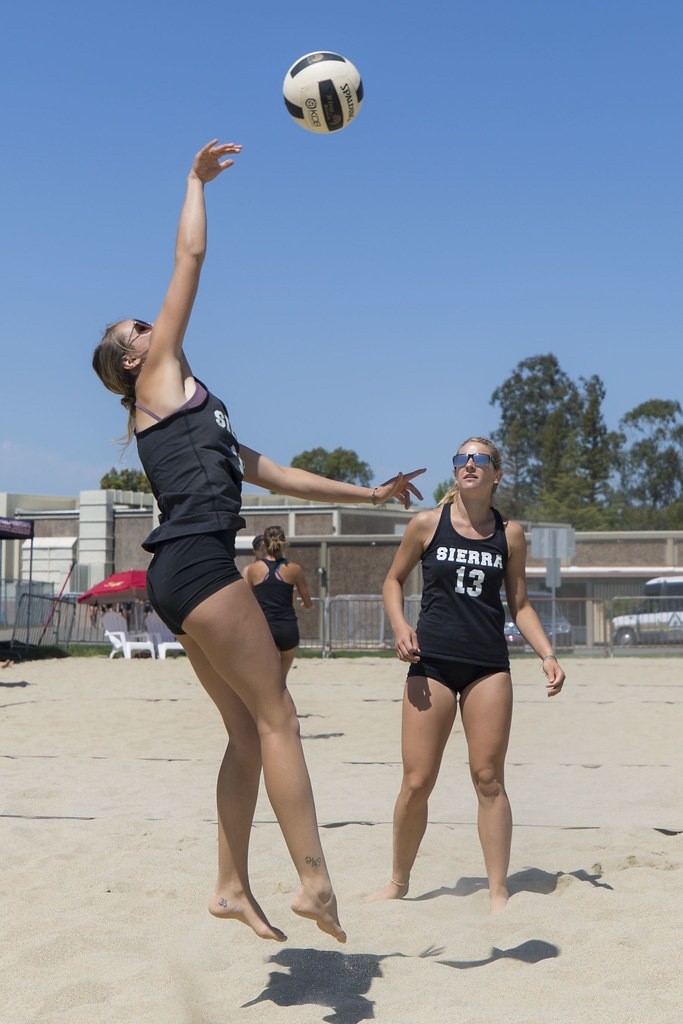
[281,50,364,136]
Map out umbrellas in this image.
[77,569,152,605]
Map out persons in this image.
[247,526,312,683]
[241,535,268,584]
[363,438,566,914]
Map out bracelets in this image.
[543,655,558,663]
[371,488,378,506]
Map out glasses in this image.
[453,454,497,467]
[127,319,153,349]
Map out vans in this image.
[610,575,683,646]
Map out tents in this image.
[91,136,426,943]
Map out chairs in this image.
[104,629,156,659]
[158,642,184,659]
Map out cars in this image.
[498,591,575,655]
